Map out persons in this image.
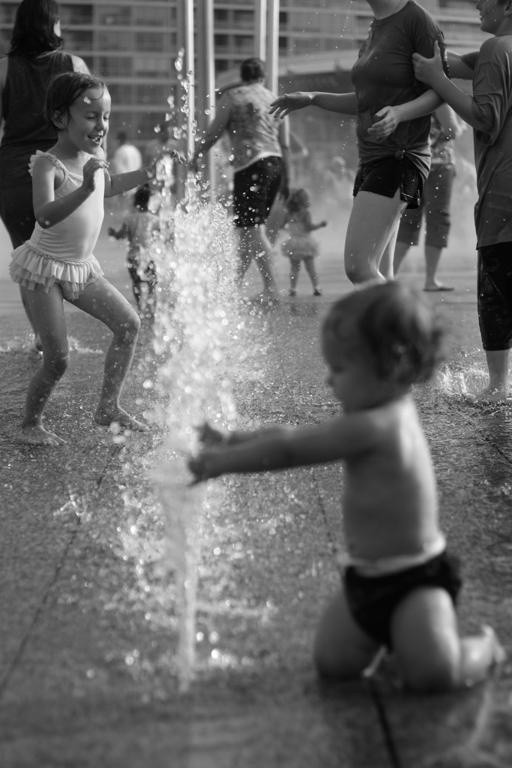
[0,0,91,347]
[392,104,463,290]
[271,2,448,281]
[189,60,354,298]
[412,1,512,402]
[108,133,165,312]
[181,278,512,691]
[9,73,160,442]
